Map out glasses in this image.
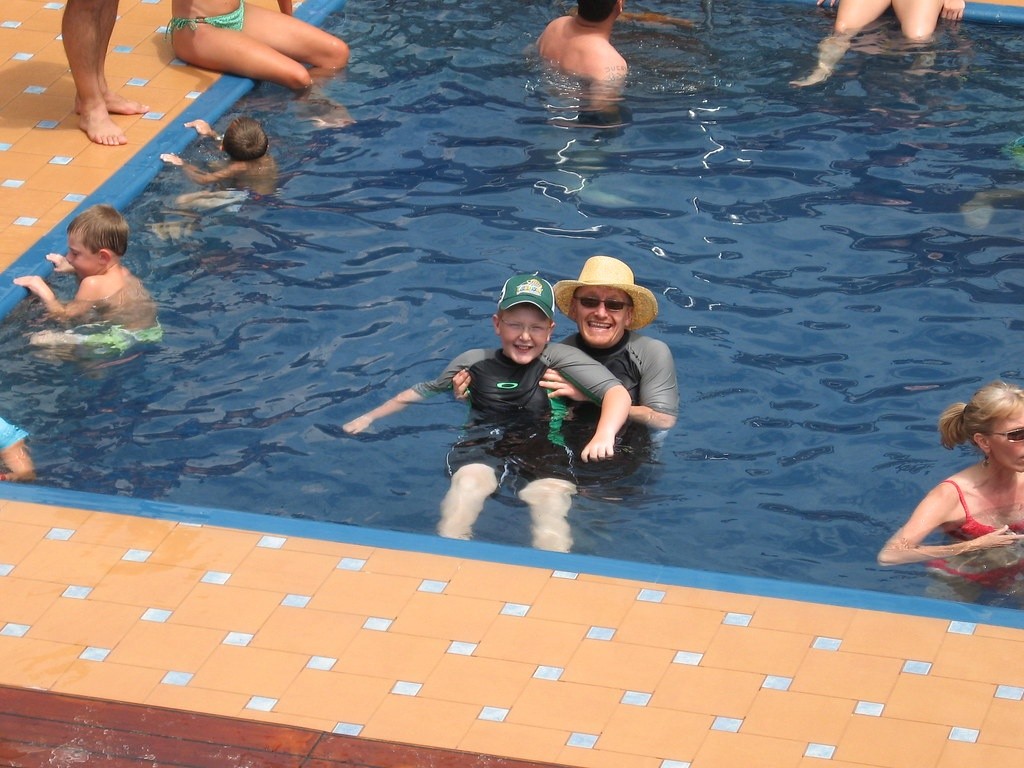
[573,296,632,311]
[991,427,1024,443]
[501,316,549,333]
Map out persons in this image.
[906,382,1024,536]
[400,273,630,457]
[538,0,627,79]
[62,0,149,146]
[165,0,349,88]
[817,0,965,38]
[452,256,679,413]
[0,417,10,439]
[13,205,130,299]
[160,118,269,165]
[563,0,664,23]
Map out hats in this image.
[552,255,658,331]
[498,274,555,319]
